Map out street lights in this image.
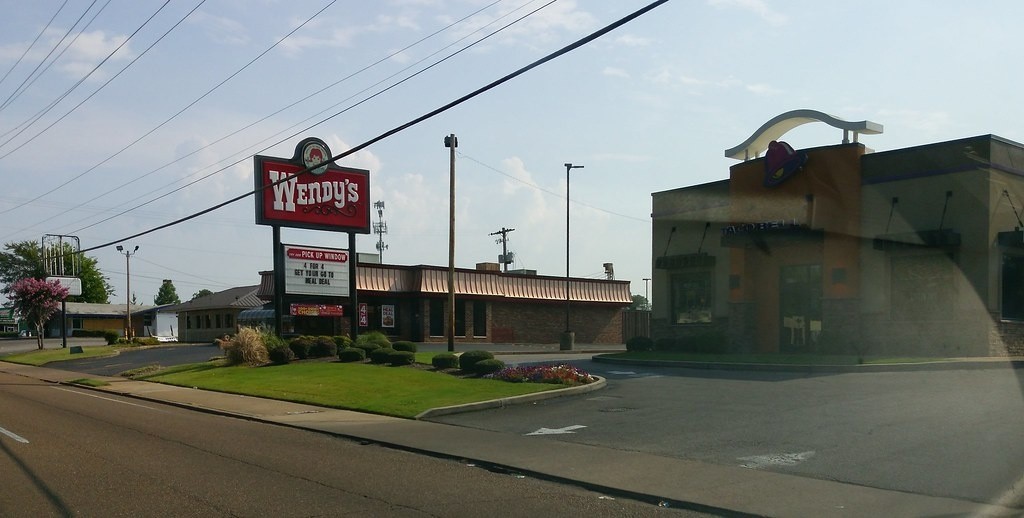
[116,245,139,345]
[565,163,586,332]
[444,133,458,352]
[643,279,651,311]
